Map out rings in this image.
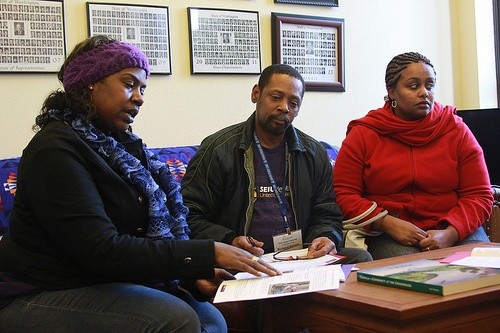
[427,247,430,251]
[252,256,259,261]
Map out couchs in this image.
[0,141,500,242]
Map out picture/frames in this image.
[271,12,346,92]
[187,6,261,75]
[0,0,66,73]
[86,1,172,75]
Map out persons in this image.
[180,64,374,275]
[193,18,258,65]
[283,30,336,81]
[334,52,493,262]
[0,34,284,333]
[93,10,168,65]
[0,4,62,63]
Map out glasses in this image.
[273,243,321,261]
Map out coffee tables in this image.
[262,241,500,333]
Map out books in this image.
[212,247,354,303]
[449,247,500,268]
[356,259,500,296]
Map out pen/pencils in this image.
[247,236,256,247]
